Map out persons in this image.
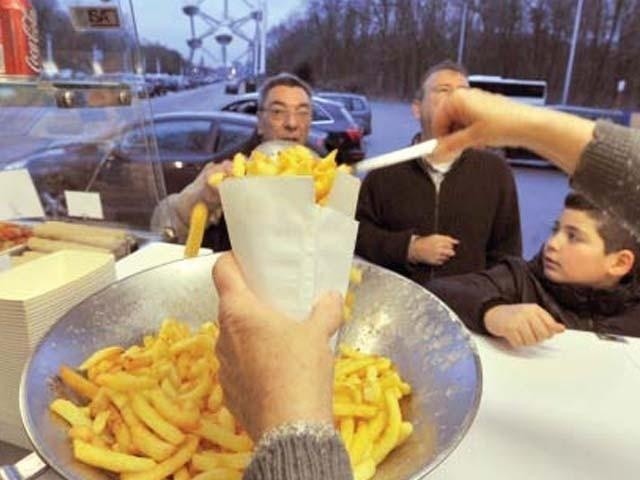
[154,71,324,240]
[212,89,640,480]
[427,192,640,348]
[353,61,522,288]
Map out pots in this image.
[16,247,479,480]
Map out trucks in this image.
[407,70,629,168]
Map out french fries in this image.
[49,320,413,480]
[183,202,210,258]
[342,266,363,320]
[207,145,353,208]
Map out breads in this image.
[11,221,132,265]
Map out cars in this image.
[218,94,364,165]
[0,63,225,109]
[0,109,339,228]
[318,93,371,140]
